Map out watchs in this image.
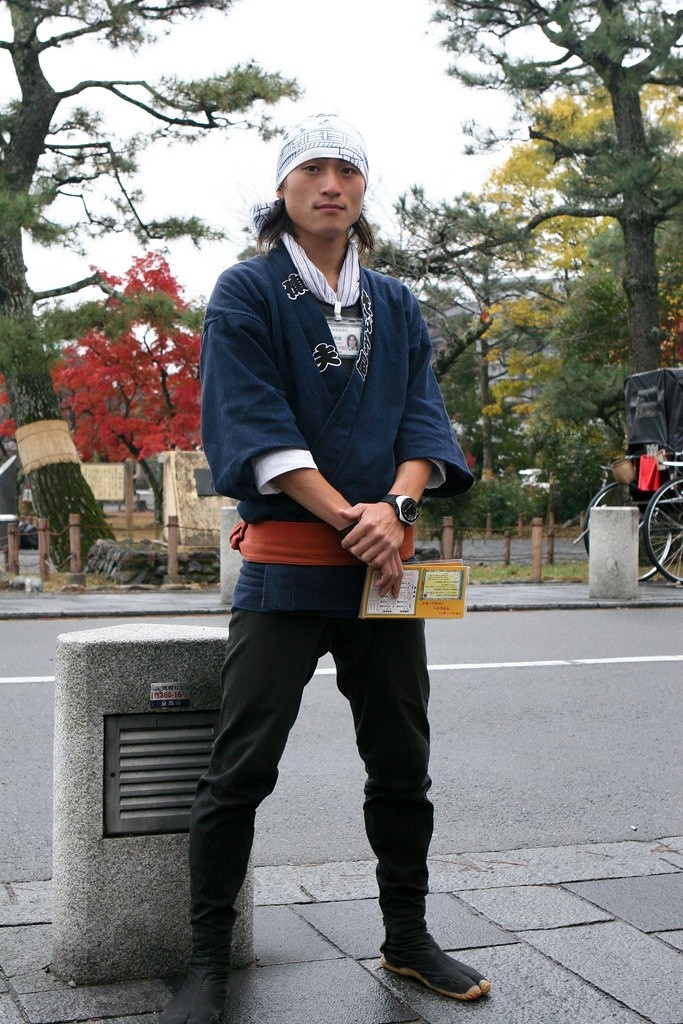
[380,493,419,527]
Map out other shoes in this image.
[159,957,230,1024]
[380,927,490,1000]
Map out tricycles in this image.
[576,366,683,587]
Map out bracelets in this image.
[339,520,360,539]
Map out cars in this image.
[520,470,551,493]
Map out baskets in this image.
[612,457,635,483]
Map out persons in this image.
[346,334,359,351]
[163,111,490,1024]
